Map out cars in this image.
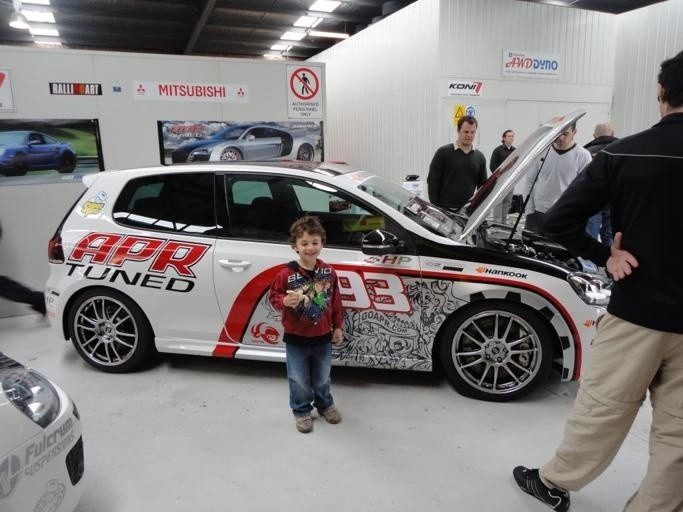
[0,349,84,510]
[46,108,606,405]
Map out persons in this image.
[426,115,487,216]
[520,119,593,236]
[0,274,47,318]
[265,215,345,434]
[580,121,620,250]
[488,129,516,226]
[510,47,682,512]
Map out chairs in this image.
[248,194,284,238]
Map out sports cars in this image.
[172,125,316,169]
[0,131,75,176]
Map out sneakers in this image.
[320,404,341,424]
[294,413,314,432]
[512,465,570,511]
